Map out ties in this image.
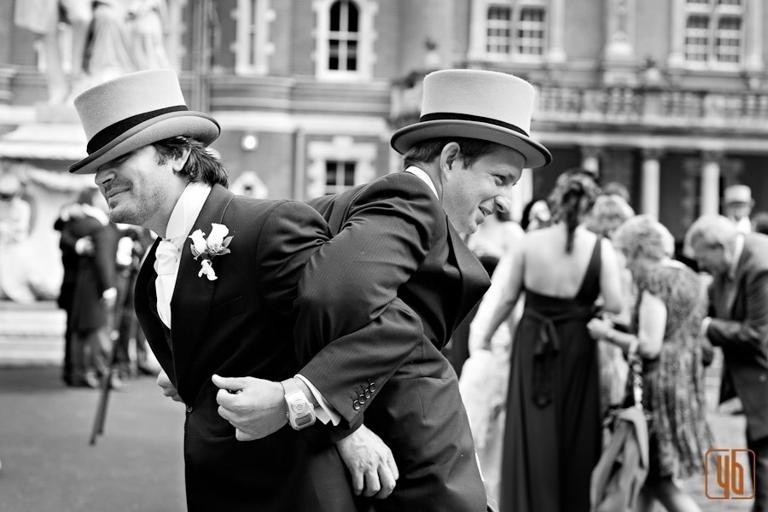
[153,240,179,330]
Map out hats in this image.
[391,69,551,169]
[69,67,221,174]
[723,185,752,204]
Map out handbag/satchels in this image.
[590,407,649,512]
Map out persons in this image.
[68,66,424,512]
[154,69,552,511]
[440,168,768,512]
[53,186,161,389]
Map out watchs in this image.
[281,378,316,432]
